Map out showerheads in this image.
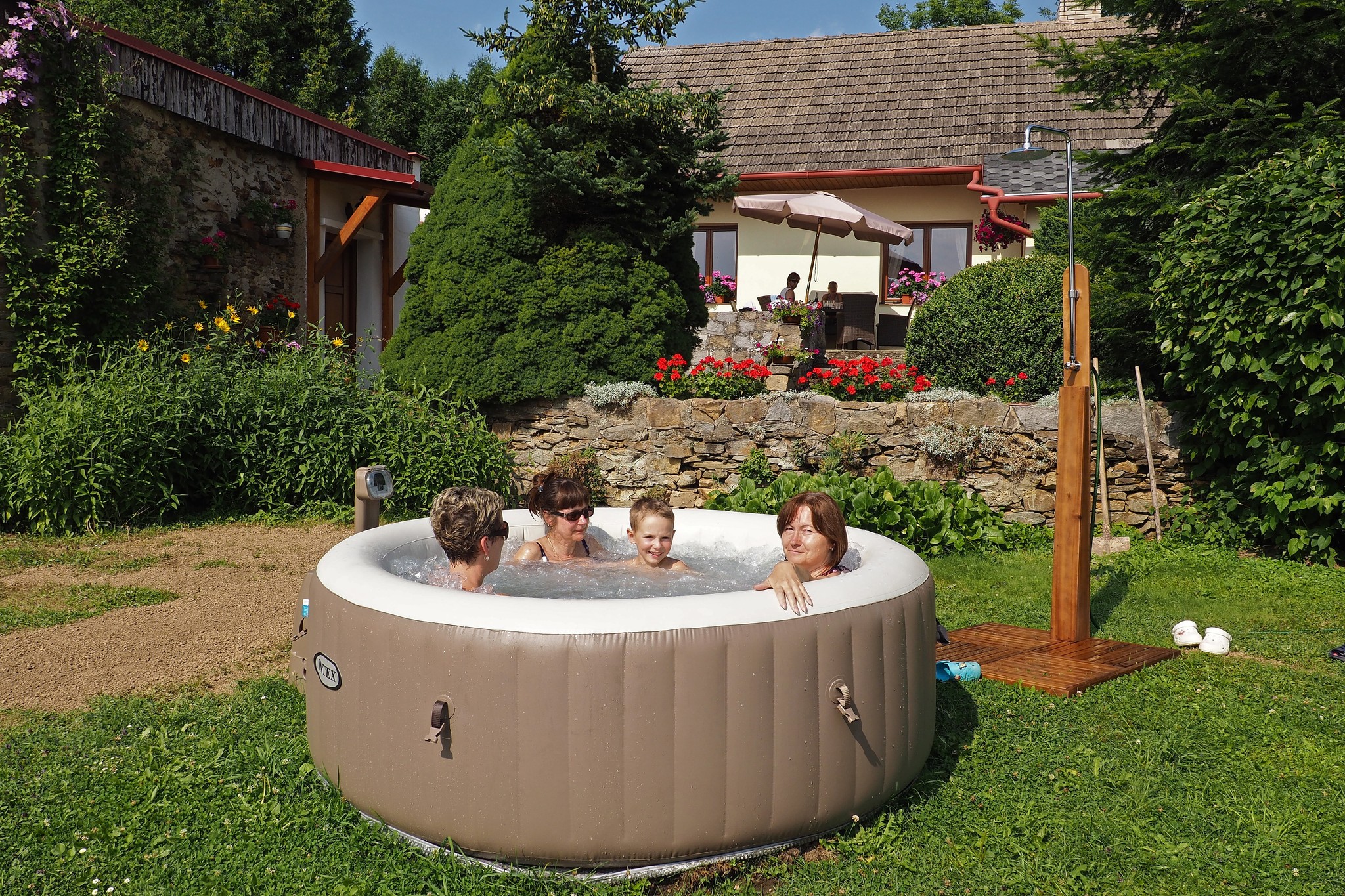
[999,125,1069,162]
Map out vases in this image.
[782,315,802,324]
[276,223,292,239]
[241,215,256,230]
[712,293,724,303]
[1008,403,1028,406]
[901,294,914,304]
[203,255,219,266]
[258,325,279,343]
[773,356,795,364]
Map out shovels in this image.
[1091,357,1130,556]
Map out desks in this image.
[822,309,843,313]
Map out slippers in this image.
[1199,626,1233,656]
[1172,621,1203,646]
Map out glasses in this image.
[483,521,509,541]
[789,280,799,283]
[550,504,595,521]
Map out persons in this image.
[752,491,848,615]
[820,280,843,333]
[778,272,801,323]
[429,471,695,597]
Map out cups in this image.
[822,300,843,309]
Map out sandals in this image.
[936,661,981,681]
[1328,644,1345,662]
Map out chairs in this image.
[876,298,918,346]
[757,295,778,311]
[836,294,878,350]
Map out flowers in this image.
[1006,372,1027,403]
[240,196,303,231]
[201,230,227,259]
[699,271,735,303]
[887,268,947,305]
[748,337,820,362]
[973,209,1030,253]
[767,295,822,336]
[260,293,300,332]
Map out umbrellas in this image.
[732,191,915,306]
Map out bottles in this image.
[814,292,819,302]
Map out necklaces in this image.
[547,533,575,561]
